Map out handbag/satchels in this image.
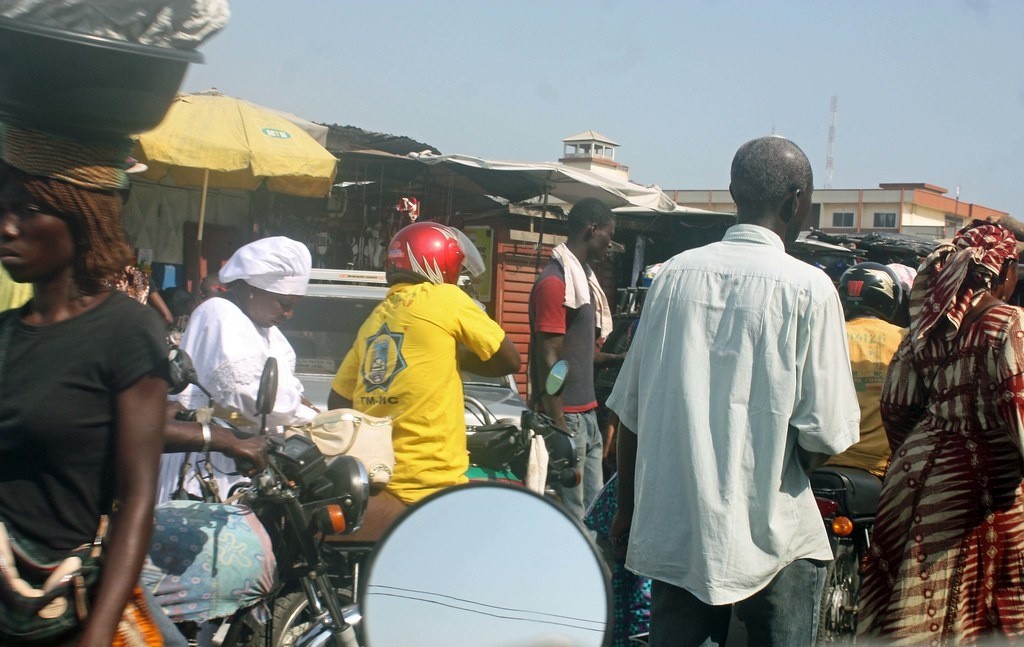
[0,515,109,639]
[284,408,394,487]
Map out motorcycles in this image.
[313,357,578,592]
[139,343,363,647]
[804,465,883,647]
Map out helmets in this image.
[838,262,903,322]
[385,220,466,285]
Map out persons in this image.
[0,123,269,647]
[328,222,521,505]
[158,236,322,505]
[606,137,861,647]
[825,218,1024,646]
[530,199,628,519]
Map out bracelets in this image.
[199,423,212,452]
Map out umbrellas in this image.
[131,87,340,281]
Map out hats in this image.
[219,235,311,295]
